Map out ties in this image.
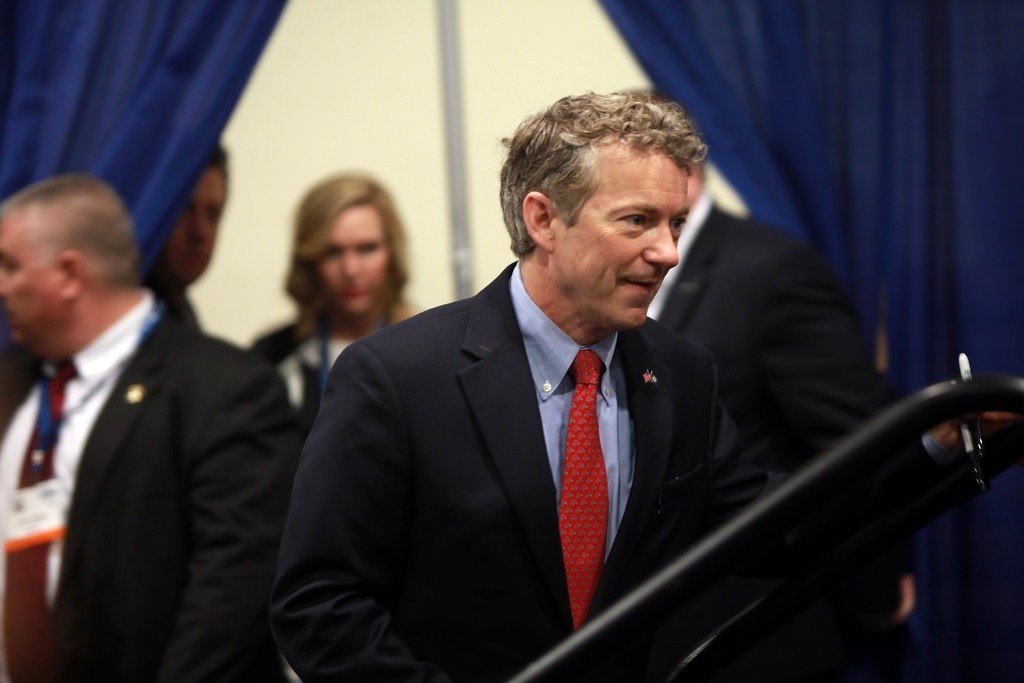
[16,352,71,620]
[557,345,611,632]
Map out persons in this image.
[140,144,228,332]
[249,174,408,444]
[267,89,1024,683]
[0,174,302,683]
